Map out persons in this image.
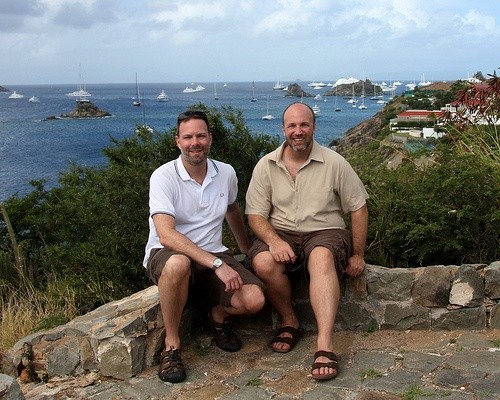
[142,111,265,382]
[244,103,369,377]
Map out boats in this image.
[312,106,320,117]
[157,90,169,102]
[405,73,431,90]
[261,94,275,119]
[9,92,23,99]
[347,80,402,110]
[183,85,205,94]
[65,89,90,96]
[27,96,40,103]
[307,82,333,90]
[133,102,141,106]
[272,80,288,91]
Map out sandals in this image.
[204,309,243,352]
[311,350,338,379]
[268,324,302,353]
[159,349,186,382]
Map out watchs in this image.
[212,258,222,271]
